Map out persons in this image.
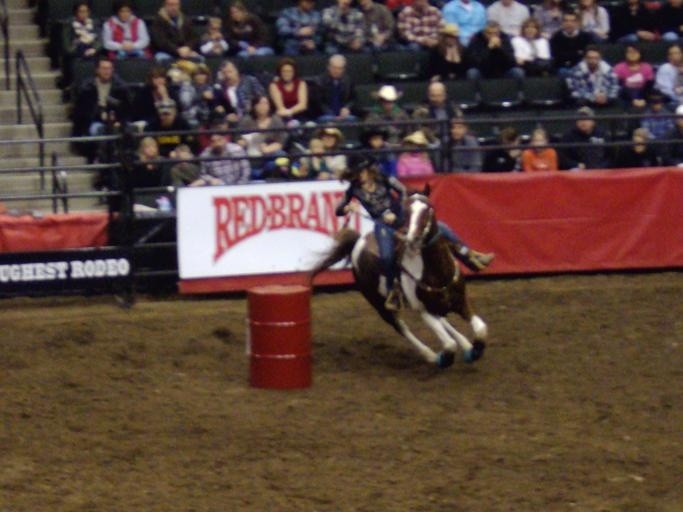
[62,1,683,203]
[328,154,495,310]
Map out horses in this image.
[304,181,488,368]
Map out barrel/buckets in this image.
[246,284,313,388]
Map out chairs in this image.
[33,2,681,184]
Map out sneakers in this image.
[386,290,398,311]
[470,252,495,273]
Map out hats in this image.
[370,85,401,102]
[437,24,459,37]
[402,130,430,146]
[315,122,343,137]
[346,153,370,171]
